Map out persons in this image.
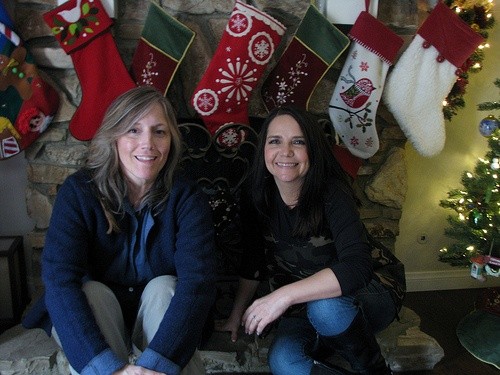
[213,107,392,375]
[21,87,212,375]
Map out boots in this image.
[318,309,391,375]
[308,357,360,375]
